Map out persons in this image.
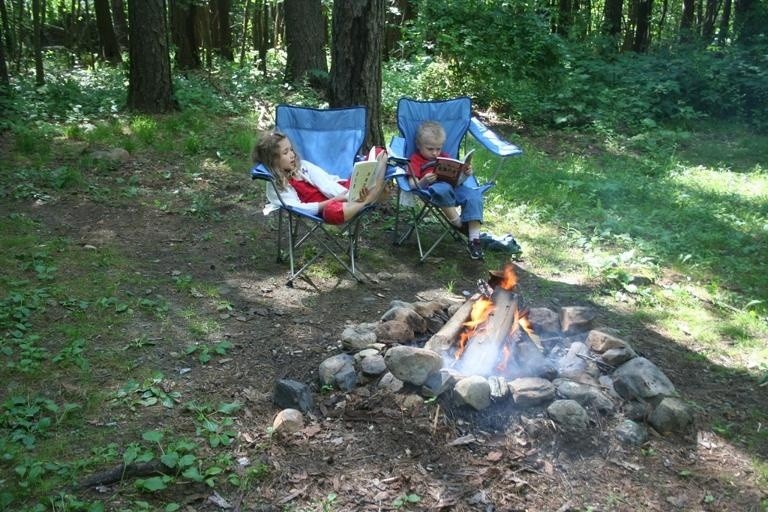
[251,133,389,225]
[407,122,485,262]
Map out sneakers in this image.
[466,238,485,260]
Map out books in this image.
[348,146,386,203]
[431,149,476,186]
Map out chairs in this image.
[386,99,522,266]
[250,99,386,287]
